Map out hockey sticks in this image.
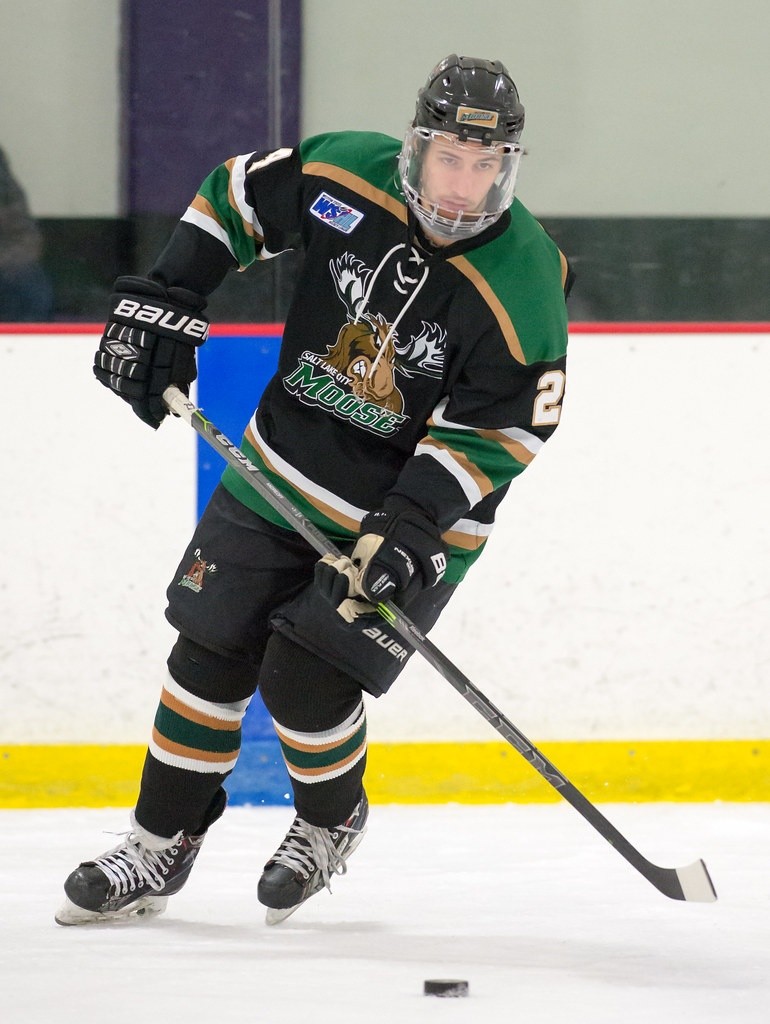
[163,381,718,903]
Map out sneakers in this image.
[257,790,368,920]
[60,796,227,923]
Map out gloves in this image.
[311,505,448,631]
[92,277,195,429]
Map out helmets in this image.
[398,52,528,238]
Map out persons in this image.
[58,54,576,923]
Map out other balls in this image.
[423,979,469,999]
